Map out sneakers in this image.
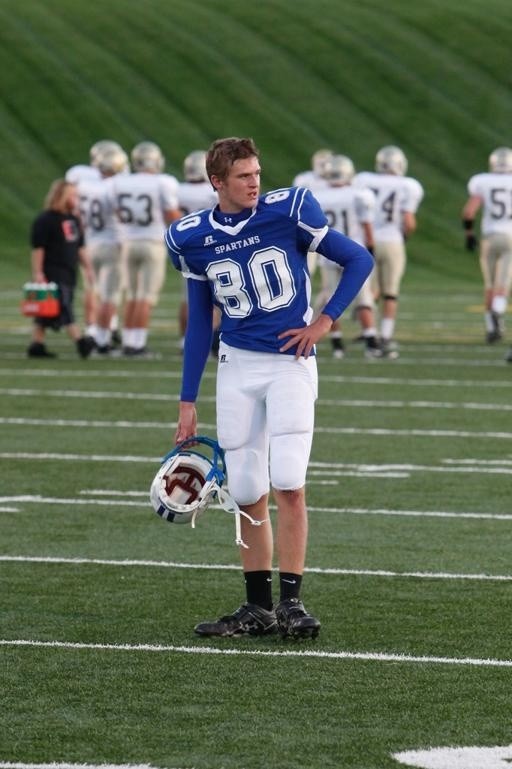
[194,602,278,638]
[78,328,160,360]
[363,341,399,361]
[275,598,321,641]
[27,343,56,357]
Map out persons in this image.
[21,138,211,360]
[290,145,423,359]
[163,135,376,640]
[457,144,511,343]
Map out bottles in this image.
[22,281,60,302]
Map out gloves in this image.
[465,234,479,251]
